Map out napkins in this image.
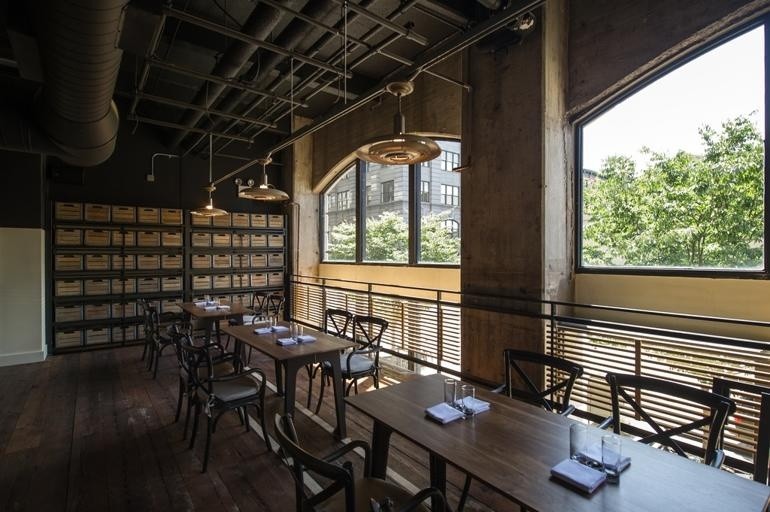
[271,325,289,333]
[275,338,295,346]
[549,457,606,496]
[298,335,316,343]
[254,327,272,335]
[426,403,464,423]
[456,395,491,414]
[580,443,633,473]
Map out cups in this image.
[599,434,623,479]
[442,377,459,410]
[265,315,304,340]
[203,294,221,310]
[567,420,592,468]
[460,384,477,418]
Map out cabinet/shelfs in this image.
[0,200,289,367]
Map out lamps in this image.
[355,77,441,167]
[190,151,290,216]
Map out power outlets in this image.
[146,175,155,182]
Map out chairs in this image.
[489,349,585,417]
[180,341,271,475]
[314,315,389,415]
[166,323,243,439]
[272,412,454,511]
[144,306,194,380]
[305,308,353,408]
[597,371,738,469]
